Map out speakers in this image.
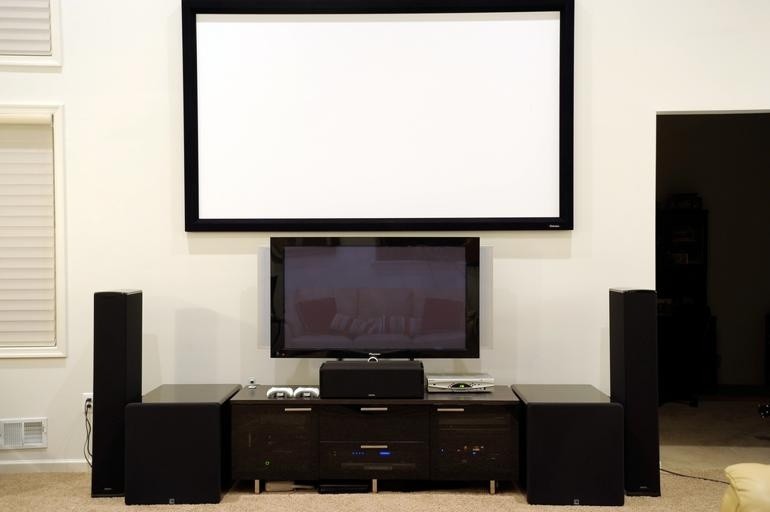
[608,288,662,498]
[90,290,143,498]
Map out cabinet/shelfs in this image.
[512,383,624,506]
[231,384,318,493]
[125,384,241,501]
[317,384,433,493]
[433,383,519,495]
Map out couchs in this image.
[337,286,415,336]
[299,299,337,348]
[411,297,466,347]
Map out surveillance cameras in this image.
[247,377,257,389]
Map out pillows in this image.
[349,318,369,334]
[368,315,388,334]
[330,314,352,333]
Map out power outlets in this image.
[83,393,93,415]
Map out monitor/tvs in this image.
[269,235,480,359]
